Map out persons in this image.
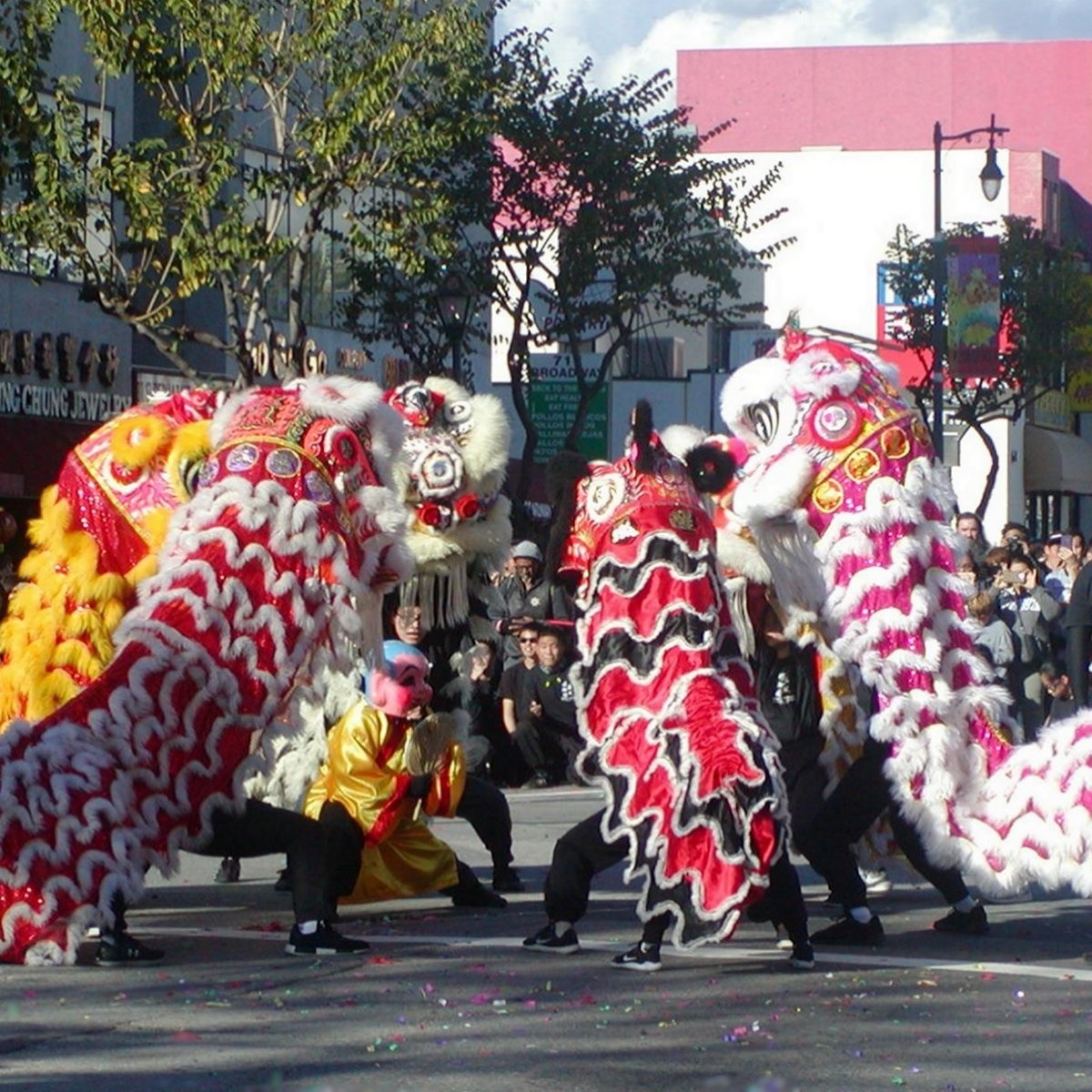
[427,637,530,790]
[609,847,815,972]
[956,510,1092,751]
[215,849,293,893]
[497,619,545,787]
[516,626,600,788]
[383,583,526,894]
[298,639,507,924]
[744,601,850,908]
[522,803,798,951]
[93,860,166,968]
[802,683,989,948]
[487,539,574,671]
[177,794,374,956]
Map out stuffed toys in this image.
[712,338,1092,901]
[1,385,233,732]
[383,374,518,636]
[563,397,795,950]
[662,419,867,798]
[3,360,413,965]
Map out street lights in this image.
[932,113,1012,458]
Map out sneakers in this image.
[934,903,991,934]
[810,914,887,947]
[609,940,663,972]
[286,919,370,957]
[788,939,816,969]
[522,922,580,954]
[94,932,166,967]
[861,868,893,896]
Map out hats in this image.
[512,540,543,564]
[1047,531,1066,545]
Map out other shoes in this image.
[822,891,843,907]
[214,856,243,884]
[274,867,291,894]
[452,886,508,909]
[775,922,795,950]
[492,866,524,892]
[529,767,552,788]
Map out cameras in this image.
[1005,534,1024,557]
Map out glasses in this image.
[519,637,537,644]
[1043,680,1063,693]
[395,611,421,625]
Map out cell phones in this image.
[1001,569,1026,583]
[1060,535,1073,549]
